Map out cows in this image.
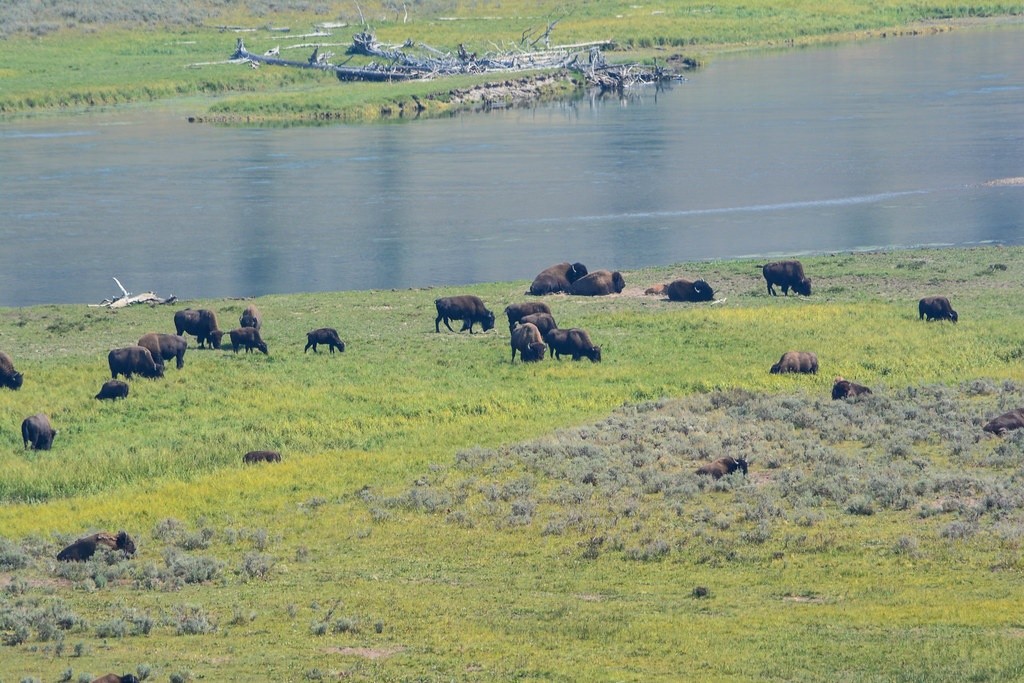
[242,451,282,464]
[831,380,873,402]
[304,327,344,354]
[56,530,137,561]
[107,304,268,380]
[21,414,57,451]
[918,296,958,321]
[0,352,23,392]
[644,277,714,302]
[433,294,495,335]
[504,301,603,363]
[755,260,812,296]
[524,262,627,297]
[983,407,1024,437]
[695,453,750,478]
[94,380,129,401]
[770,351,818,375]
